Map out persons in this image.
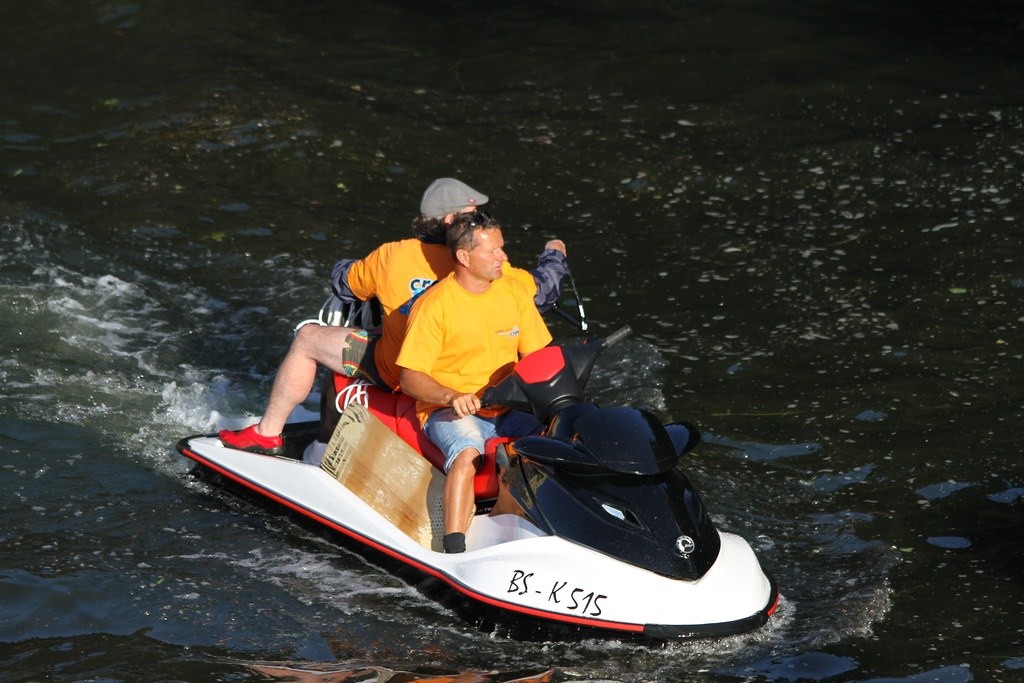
[218,178,566,455]
[396,212,554,553]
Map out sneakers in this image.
[218,424,285,455]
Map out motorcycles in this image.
[177,324,780,649]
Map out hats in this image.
[419,177,489,216]
